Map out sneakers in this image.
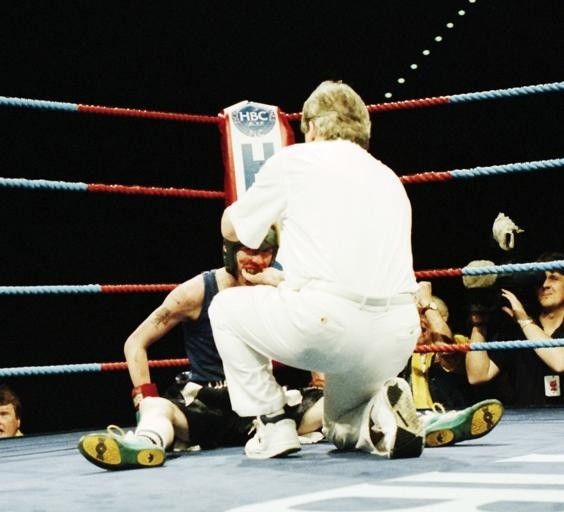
[422,397,506,450]
[77,423,167,471]
[244,416,302,461]
[374,375,426,460]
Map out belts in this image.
[309,281,415,308]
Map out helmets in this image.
[222,228,280,280]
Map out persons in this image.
[398,250,564,409]
[0,385,24,438]
[207,82,426,459]
[79,227,505,470]
